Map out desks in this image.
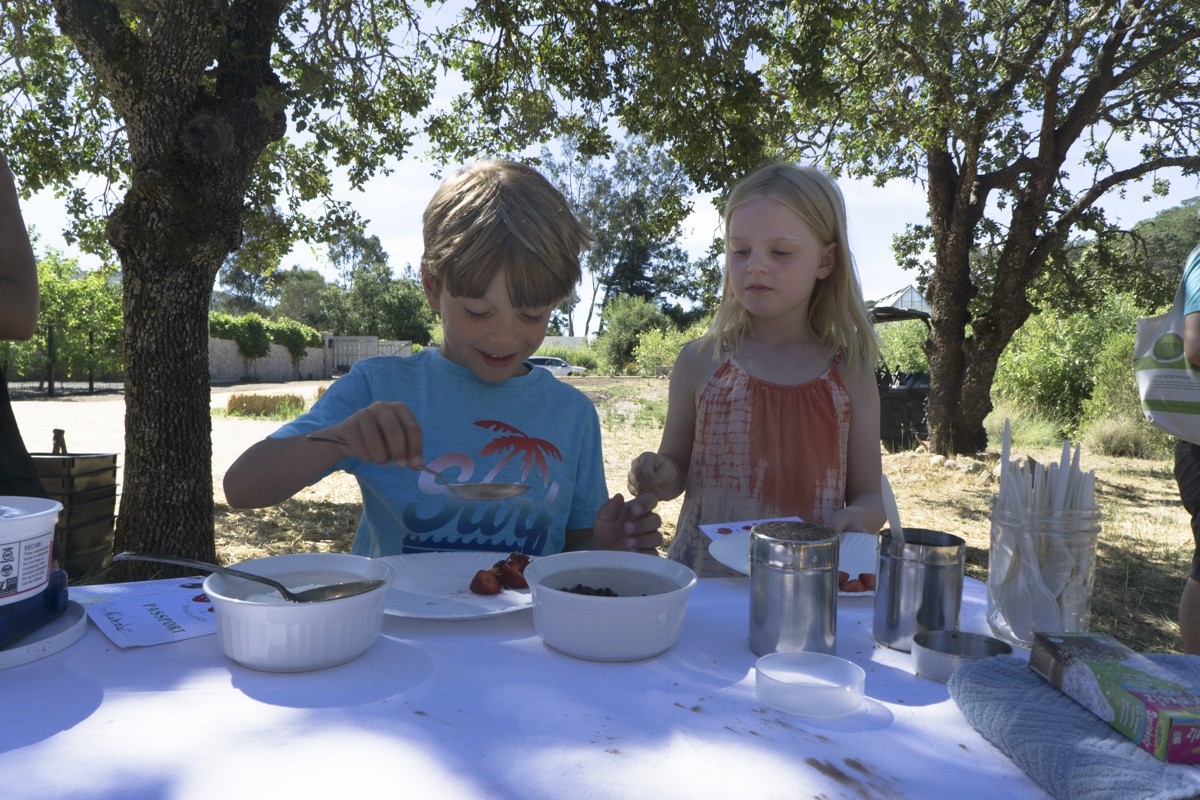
[0,578,1200,800]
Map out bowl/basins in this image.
[202,553,396,674]
[523,550,700,662]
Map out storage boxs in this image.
[1030,632,1200,763]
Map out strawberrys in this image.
[470,553,533,595]
[839,571,876,592]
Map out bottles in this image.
[748,520,841,657]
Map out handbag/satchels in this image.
[1132,250,1200,446]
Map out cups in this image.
[989,498,1103,649]
[0,495,63,606]
[874,527,967,652]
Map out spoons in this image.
[304,433,531,502]
[112,550,385,602]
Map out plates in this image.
[373,551,545,620]
[707,532,876,596]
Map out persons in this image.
[1172,246,1200,655]
[0,153,51,498]
[223,159,663,557]
[627,164,886,579]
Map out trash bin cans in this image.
[29,451,122,577]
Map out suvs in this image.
[527,356,588,378]
